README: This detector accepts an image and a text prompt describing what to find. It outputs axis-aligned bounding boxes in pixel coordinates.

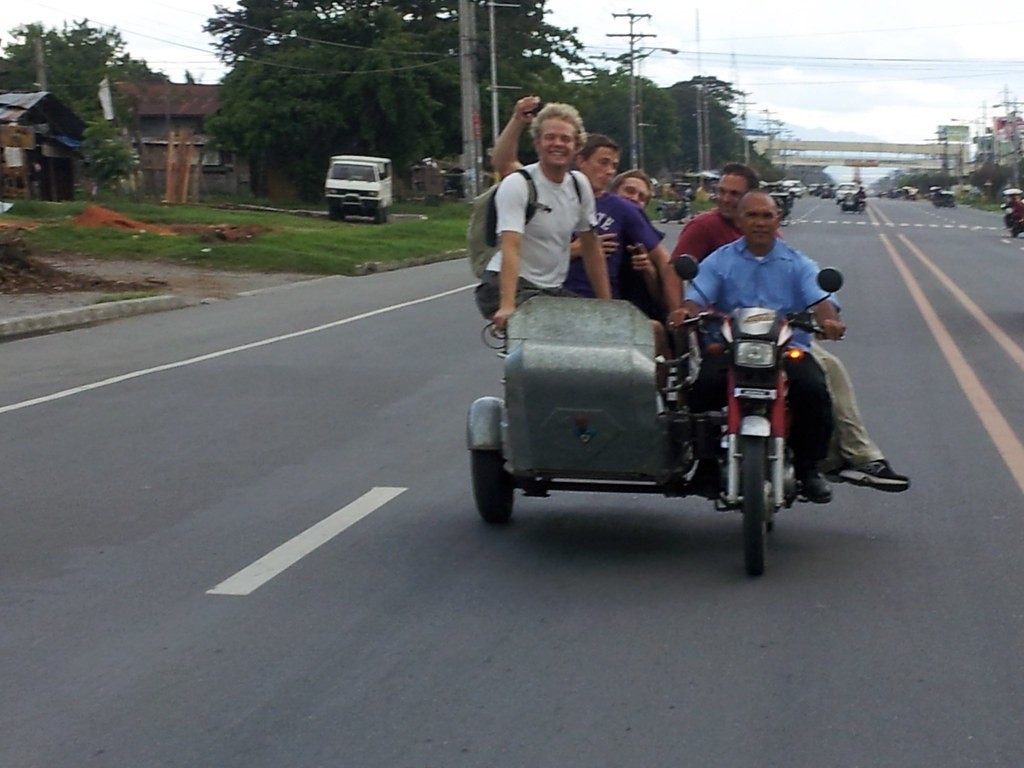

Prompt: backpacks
[467,169,582,280]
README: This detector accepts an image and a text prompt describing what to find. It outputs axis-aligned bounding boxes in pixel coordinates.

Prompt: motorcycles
[930,187,954,208]
[1000,190,1024,236]
[468,254,848,578]
[657,189,694,226]
[840,186,867,214]
[771,193,791,227]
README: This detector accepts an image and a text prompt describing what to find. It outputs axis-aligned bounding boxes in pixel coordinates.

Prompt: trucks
[326,156,392,223]
[682,173,719,203]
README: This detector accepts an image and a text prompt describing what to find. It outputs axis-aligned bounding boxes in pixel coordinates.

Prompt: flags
[997,116,1024,129]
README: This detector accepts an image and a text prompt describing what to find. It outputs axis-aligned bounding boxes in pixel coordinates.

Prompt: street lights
[636,48,679,170]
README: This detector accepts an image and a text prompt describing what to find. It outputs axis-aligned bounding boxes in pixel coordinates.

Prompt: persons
[1004,196,1024,227]
[569,170,662,296]
[668,164,914,486]
[489,99,682,314]
[665,191,846,505]
[475,104,665,414]
[853,186,866,203]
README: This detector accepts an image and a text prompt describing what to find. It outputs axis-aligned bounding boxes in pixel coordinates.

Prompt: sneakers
[838,458,911,493]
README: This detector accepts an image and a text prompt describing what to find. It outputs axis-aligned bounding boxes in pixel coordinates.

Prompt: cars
[788,186,802,197]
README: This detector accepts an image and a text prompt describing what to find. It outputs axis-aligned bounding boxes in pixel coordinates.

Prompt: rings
[669,321,674,325]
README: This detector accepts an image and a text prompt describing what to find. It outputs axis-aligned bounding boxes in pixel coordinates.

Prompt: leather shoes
[801,468,833,505]
